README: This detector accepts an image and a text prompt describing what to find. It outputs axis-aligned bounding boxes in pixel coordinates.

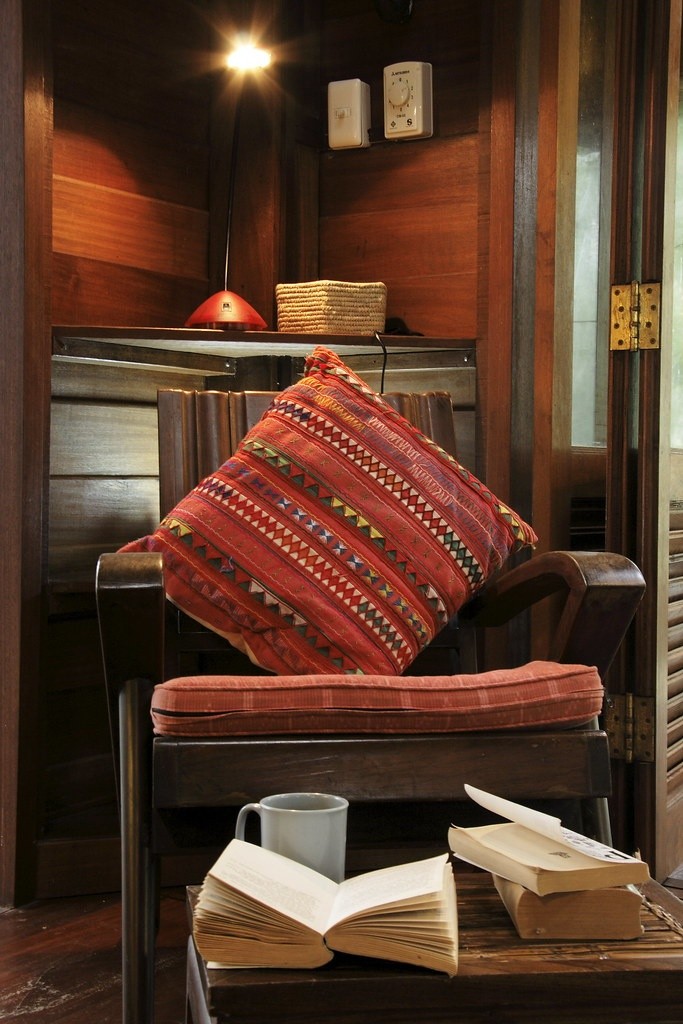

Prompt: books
[490,872,644,939]
[192,839,458,978]
[447,783,650,897]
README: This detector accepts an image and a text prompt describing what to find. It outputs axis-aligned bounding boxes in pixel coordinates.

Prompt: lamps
[178,20,284,334]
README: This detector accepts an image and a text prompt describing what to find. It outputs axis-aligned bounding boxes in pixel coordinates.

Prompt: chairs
[95,389,683,1024]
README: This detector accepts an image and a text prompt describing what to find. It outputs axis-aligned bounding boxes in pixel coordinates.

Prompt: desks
[184,861,683,1024]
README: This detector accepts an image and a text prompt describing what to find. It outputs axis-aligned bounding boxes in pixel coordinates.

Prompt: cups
[234,791,349,884]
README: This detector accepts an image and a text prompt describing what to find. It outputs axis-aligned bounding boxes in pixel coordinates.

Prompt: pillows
[116,344,542,682]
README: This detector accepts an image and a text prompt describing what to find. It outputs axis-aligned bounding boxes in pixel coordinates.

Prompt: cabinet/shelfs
[13,320,483,907]
